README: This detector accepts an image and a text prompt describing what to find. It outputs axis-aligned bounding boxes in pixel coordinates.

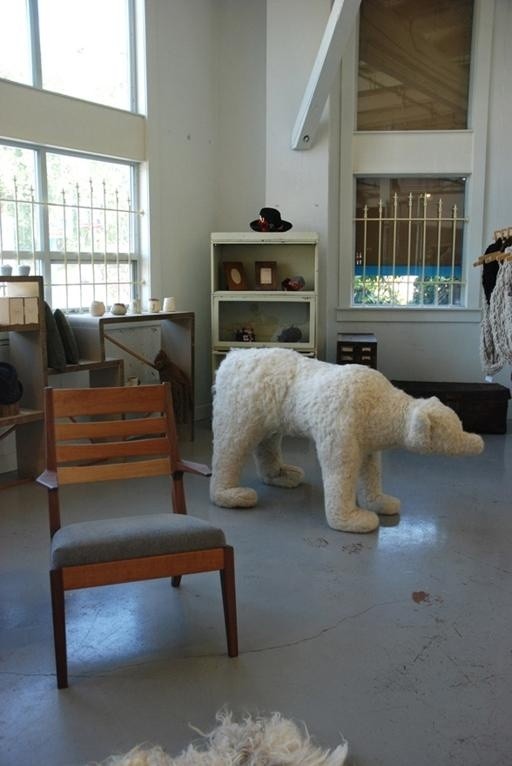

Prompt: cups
[147,298,161,313]
[129,299,141,314]
[110,302,128,316]
[88,301,106,318]
[0,264,13,276]
[17,266,31,276]
[128,376,138,387]
[163,297,177,313]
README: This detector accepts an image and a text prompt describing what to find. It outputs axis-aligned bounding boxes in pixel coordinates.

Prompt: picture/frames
[223,259,277,292]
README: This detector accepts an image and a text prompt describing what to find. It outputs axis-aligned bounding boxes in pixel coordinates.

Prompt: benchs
[383,382,512,438]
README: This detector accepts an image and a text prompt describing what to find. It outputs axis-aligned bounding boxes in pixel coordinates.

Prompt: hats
[249,207,293,232]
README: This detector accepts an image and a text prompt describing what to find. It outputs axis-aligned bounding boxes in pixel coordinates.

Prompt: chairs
[41,380,242,688]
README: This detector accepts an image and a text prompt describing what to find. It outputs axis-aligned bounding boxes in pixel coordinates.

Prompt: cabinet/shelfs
[0,274,198,494]
[208,229,322,402]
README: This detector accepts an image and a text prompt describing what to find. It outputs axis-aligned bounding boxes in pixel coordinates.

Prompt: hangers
[473,224,511,269]
